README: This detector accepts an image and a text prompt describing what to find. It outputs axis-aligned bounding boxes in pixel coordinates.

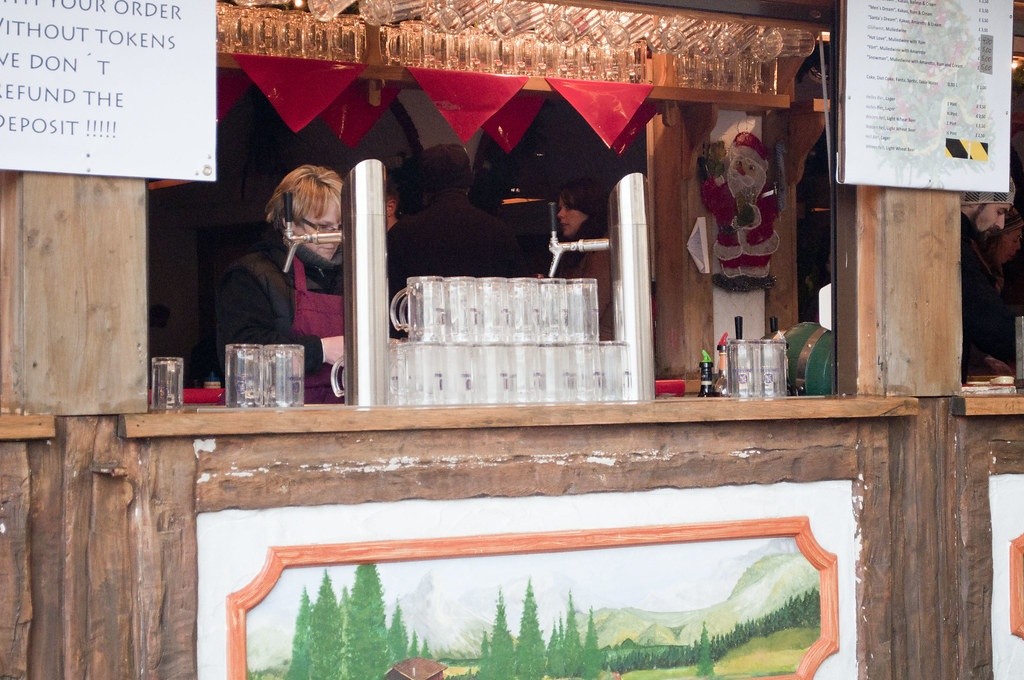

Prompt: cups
[661,15,709,55]
[553,8,601,47]
[445,276,476,341]
[358,0,426,27]
[379,20,647,84]
[493,1,558,41]
[729,339,787,397]
[507,277,539,342]
[264,344,304,407]
[693,22,734,58]
[330,341,629,404]
[762,27,814,57]
[539,278,565,342]
[566,278,599,341]
[152,357,184,410]
[307,0,358,22]
[216,2,366,64]
[672,55,764,95]
[477,277,511,341]
[712,23,760,60]
[655,379,686,397]
[225,344,263,408]
[606,11,655,50]
[390,276,446,341]
[438,0,505,35]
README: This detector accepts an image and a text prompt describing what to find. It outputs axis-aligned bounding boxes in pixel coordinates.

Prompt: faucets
[546,201,611,278]
[281,190,343,274]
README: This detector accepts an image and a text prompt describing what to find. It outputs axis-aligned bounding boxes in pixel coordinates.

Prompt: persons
[536,179,615,341]
[961,176,1015,384]
[976,205,1024,299]
[215,165,345,405]
[385,142,533,339]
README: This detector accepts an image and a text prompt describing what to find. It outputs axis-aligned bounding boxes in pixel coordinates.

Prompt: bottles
[760,316,786,339]
[713,345,728,397]
[698,362,714,397]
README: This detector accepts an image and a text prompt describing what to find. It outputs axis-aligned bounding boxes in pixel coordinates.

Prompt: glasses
[293,215,342,234]
